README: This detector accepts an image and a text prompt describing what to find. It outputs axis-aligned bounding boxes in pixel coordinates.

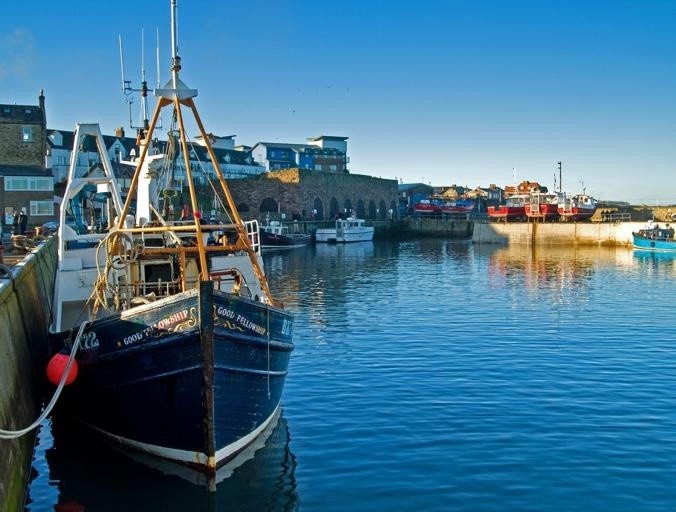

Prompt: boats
[631,248,676,263]
[414,189,601,221]
[629,213,676,250]
[26,425,305,512]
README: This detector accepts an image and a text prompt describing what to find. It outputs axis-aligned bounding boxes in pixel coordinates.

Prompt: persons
[11,210,19,236]
[18,211,28,233]
